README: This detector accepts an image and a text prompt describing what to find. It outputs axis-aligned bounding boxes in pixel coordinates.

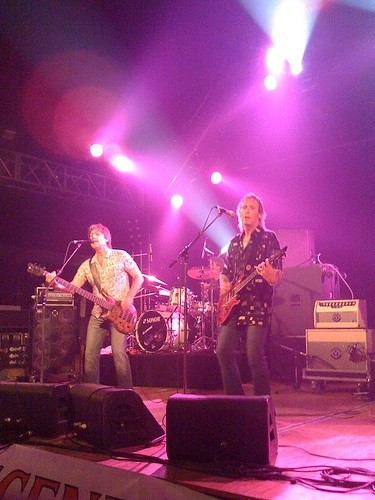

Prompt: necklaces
[243,237,248,248]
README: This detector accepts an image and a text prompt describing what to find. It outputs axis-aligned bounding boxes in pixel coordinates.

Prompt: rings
[259,268,262,271]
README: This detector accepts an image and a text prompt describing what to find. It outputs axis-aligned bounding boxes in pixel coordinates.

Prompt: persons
[45,224,145,388]
[220,193,283,416]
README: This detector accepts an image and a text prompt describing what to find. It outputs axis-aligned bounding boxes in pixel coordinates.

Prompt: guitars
[217,245,289,325]
[27,263,138,334]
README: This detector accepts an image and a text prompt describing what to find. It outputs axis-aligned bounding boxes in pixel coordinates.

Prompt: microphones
[74,239,93,243]
[149,244,153,261]
[215,204,235,217]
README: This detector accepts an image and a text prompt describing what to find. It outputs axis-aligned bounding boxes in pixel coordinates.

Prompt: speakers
[70,381,165,452]
[0,381,73,436]
[166,393,276,469]
[31,302,77,380]
[307,328,375,371]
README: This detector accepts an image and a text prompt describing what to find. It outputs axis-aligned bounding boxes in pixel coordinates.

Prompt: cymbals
[143,275,167,286]
[188,266,216,280]
[150,284,170,292]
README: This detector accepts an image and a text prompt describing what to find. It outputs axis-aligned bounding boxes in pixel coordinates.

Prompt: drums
[134,310,189,354]
[167,286,193,312]
[190,300,212,315]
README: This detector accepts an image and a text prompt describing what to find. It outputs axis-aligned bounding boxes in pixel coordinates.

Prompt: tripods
[188,281,218,353]
